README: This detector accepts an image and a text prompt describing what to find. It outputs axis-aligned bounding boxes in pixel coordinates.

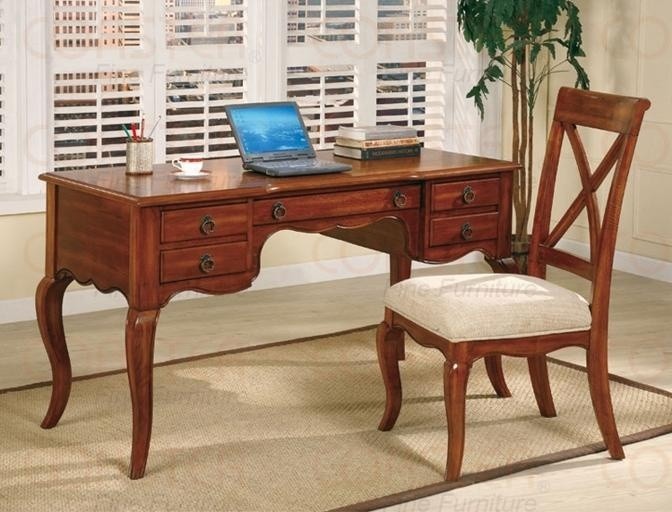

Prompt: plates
[172,171,210,180]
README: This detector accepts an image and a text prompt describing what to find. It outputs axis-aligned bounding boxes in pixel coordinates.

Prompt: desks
[37,145,525,479]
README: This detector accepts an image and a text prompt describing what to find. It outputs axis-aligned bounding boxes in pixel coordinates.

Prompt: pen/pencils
[122,113,161,142]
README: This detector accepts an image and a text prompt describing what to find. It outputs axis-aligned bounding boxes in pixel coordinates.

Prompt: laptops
[224,101,352,177]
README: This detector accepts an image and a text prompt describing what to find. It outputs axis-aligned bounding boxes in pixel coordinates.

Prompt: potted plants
[457,0,590,274]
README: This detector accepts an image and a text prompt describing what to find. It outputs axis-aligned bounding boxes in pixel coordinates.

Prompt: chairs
[376,88,650,484]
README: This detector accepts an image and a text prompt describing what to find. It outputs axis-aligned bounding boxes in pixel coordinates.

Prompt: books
[332,144,420,160]
[335,136,418,149]
[339,123,417,141]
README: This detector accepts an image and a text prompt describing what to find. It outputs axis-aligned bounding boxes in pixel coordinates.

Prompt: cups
[172,157,203,174]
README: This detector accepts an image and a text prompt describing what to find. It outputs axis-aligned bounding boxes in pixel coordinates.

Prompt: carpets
[0,324,672,512]
